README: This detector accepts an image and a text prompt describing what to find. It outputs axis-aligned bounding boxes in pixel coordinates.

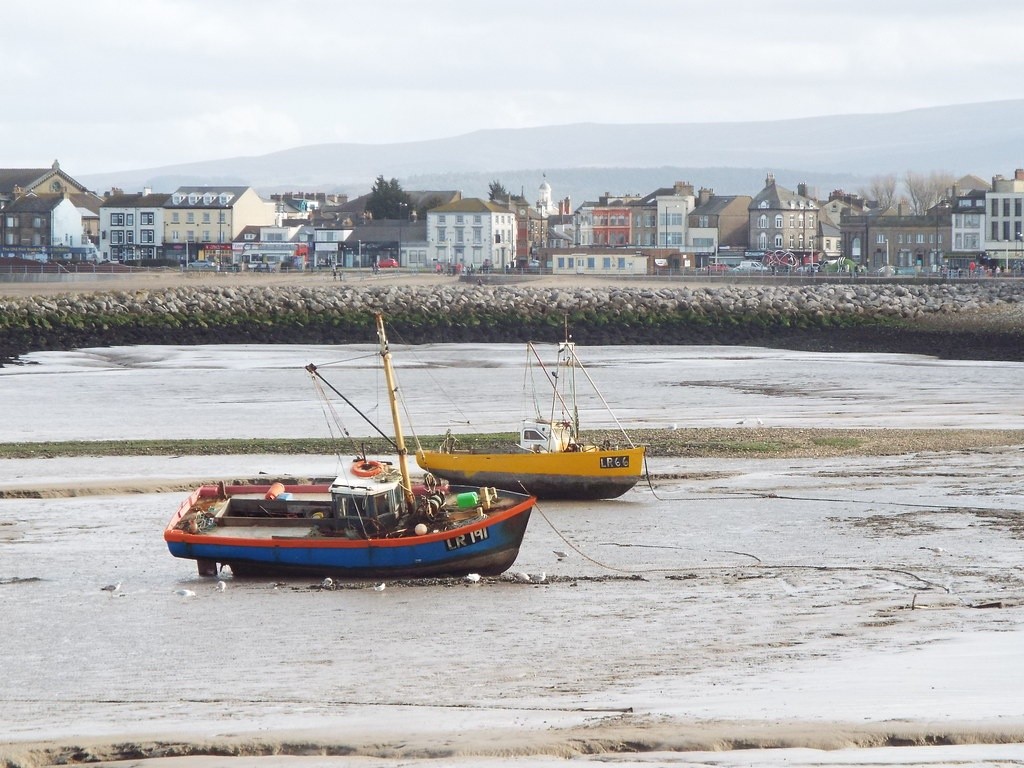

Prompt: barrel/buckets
[457,492,478,509]
[266,483,285,501]
[277,493,293,500]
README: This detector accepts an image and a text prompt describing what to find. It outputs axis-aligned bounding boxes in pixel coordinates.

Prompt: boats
[415,299,646,500]
[164,311,533,579]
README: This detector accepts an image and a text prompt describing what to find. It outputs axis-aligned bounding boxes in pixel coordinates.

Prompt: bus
[239,241,310,271]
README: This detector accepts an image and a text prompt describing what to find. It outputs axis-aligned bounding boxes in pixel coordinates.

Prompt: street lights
[393,202,408,268]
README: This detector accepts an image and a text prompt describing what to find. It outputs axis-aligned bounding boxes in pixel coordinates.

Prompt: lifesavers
[350,461,383,476]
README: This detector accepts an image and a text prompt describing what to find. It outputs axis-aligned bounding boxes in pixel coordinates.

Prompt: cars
[733,261,771,272]
[376,257,399,268]
[705,262,731,271]
